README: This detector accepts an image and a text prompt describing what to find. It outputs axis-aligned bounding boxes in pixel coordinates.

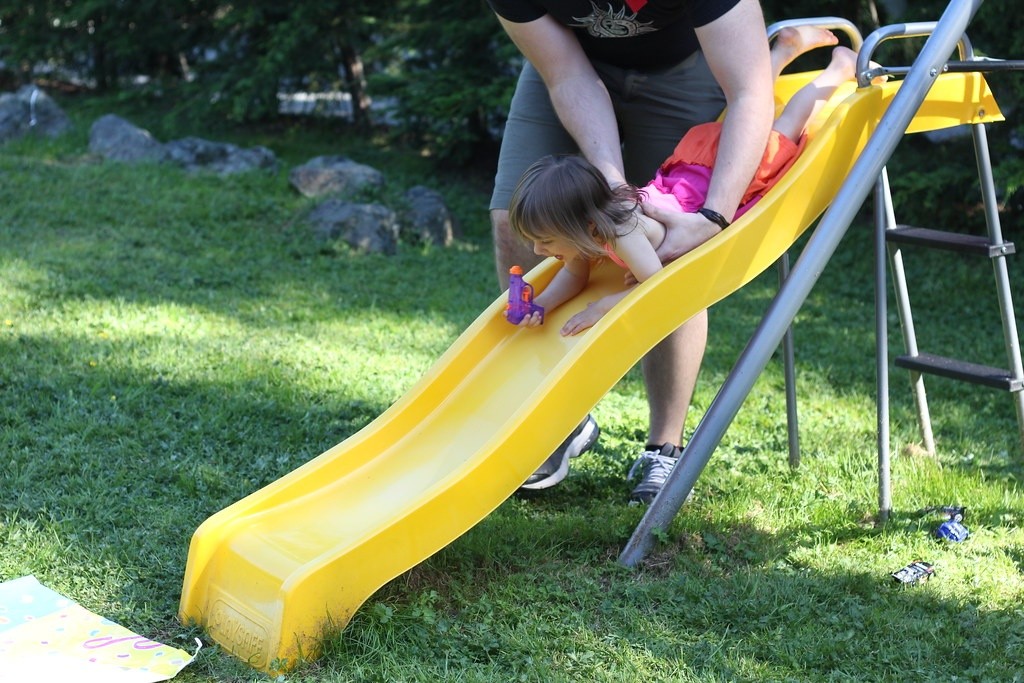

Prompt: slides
[176,65,1007,675]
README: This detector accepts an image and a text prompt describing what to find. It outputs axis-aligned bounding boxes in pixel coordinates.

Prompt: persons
[516,25,888,337]
[490,0,775,507]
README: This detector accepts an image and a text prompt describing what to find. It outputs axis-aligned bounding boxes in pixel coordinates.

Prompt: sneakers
[519,411,602,491]
[626,441,696,507]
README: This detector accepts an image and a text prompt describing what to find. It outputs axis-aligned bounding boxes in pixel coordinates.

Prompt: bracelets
[697,208,730,230]
